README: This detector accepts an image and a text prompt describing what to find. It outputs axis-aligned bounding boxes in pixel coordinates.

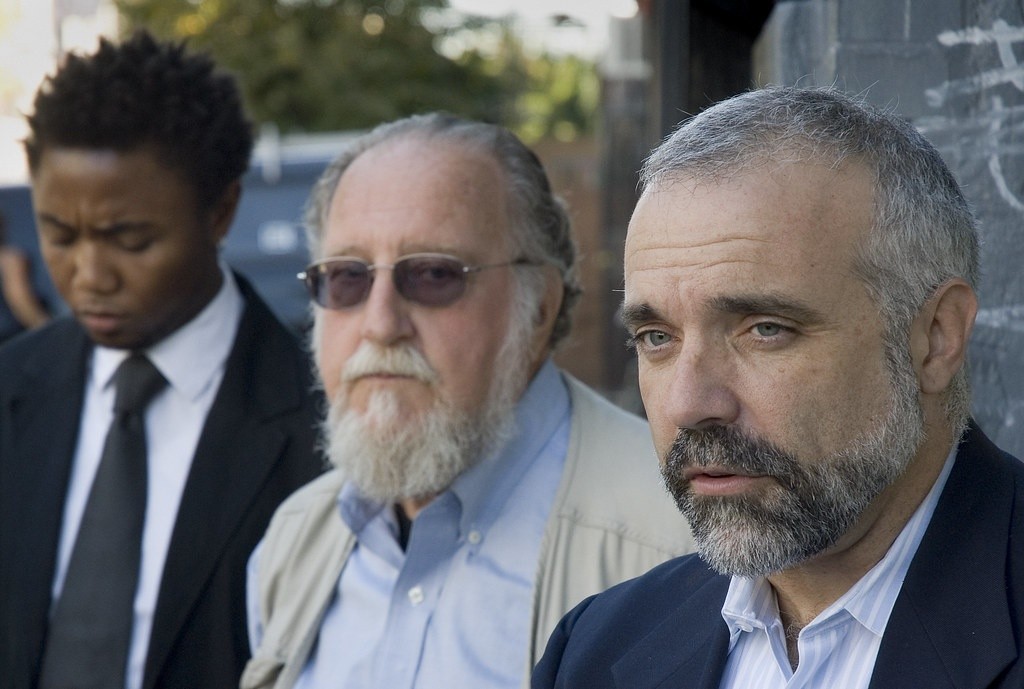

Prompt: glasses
[300,254,529,310]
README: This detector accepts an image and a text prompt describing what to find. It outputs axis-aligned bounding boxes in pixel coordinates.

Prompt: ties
[37,351,169,689]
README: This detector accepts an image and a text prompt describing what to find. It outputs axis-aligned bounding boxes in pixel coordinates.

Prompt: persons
[532,87,1024,689]
[0,30,332,689]
[240,111,700,689]
